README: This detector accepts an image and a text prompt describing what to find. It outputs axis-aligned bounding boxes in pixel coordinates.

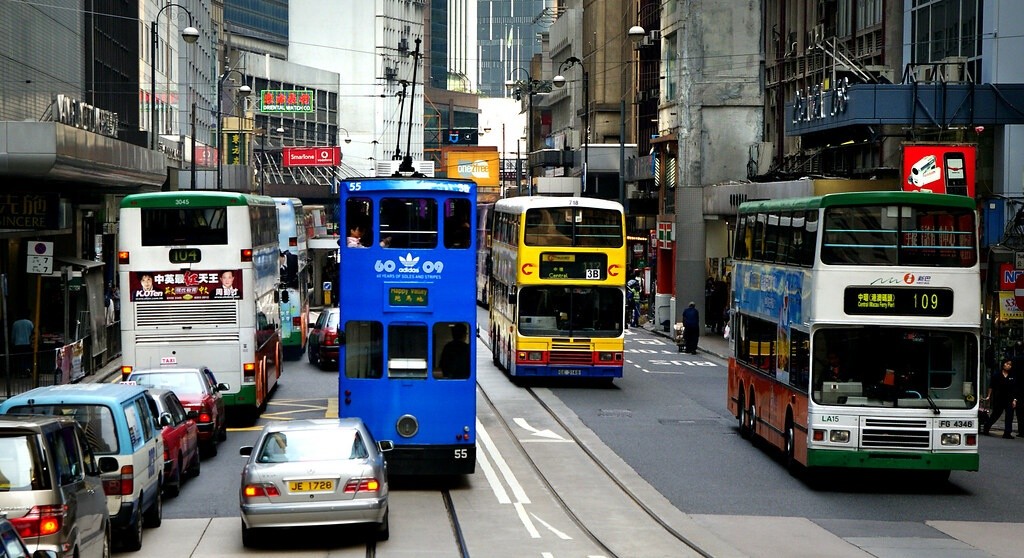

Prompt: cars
[307,307,340,370]
[146,388,202,500]
[127,365,230,459]
[0,514,59,558]
[238,418,395,552]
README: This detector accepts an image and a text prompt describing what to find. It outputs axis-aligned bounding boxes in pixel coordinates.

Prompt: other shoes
[692,351,697,355]
[636,325,643,328]
[26,369,31,377]
[684,349,691,353]
[1002,430,1014,439]
[630,324,635,327]
[984,424,991,436]
[21,374,28,377]
[1016,433,1024,436]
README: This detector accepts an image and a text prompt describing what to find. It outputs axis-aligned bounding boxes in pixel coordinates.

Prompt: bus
[727,192,982,487]
[489,195,626,388]
[118,191,288,426]
[206,197,314,360]
[338,176,478,472]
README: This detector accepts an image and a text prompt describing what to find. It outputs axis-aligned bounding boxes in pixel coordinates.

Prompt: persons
[439,324,470,379]
[983,357,1024,439]
[683,301,699,355]
[11,319,34,377]
[705,276,729,332]
[105,284,120,326]
[347,223,392,248]
[133,272,167,301]
[209,270,242,299]
[626,286,644,328]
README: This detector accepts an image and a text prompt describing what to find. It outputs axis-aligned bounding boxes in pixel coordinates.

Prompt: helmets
[628,279,636,286]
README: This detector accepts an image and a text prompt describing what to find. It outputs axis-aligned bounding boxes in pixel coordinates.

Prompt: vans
[0,413,119,558]
[0,384,173,553]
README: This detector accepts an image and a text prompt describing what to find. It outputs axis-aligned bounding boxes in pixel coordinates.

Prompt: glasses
[465,333,468,335]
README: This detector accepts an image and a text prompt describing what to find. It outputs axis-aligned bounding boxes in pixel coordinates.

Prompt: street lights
[505,68,533,153]
[151,4,200,150]
[261,117,285,195]
[217,70,252,191]
[553,56,589,196]
[332,128,351,192]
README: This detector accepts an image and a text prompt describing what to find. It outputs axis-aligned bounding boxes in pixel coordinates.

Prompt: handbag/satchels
[725,325,730,339]
[979,399,990,424]
